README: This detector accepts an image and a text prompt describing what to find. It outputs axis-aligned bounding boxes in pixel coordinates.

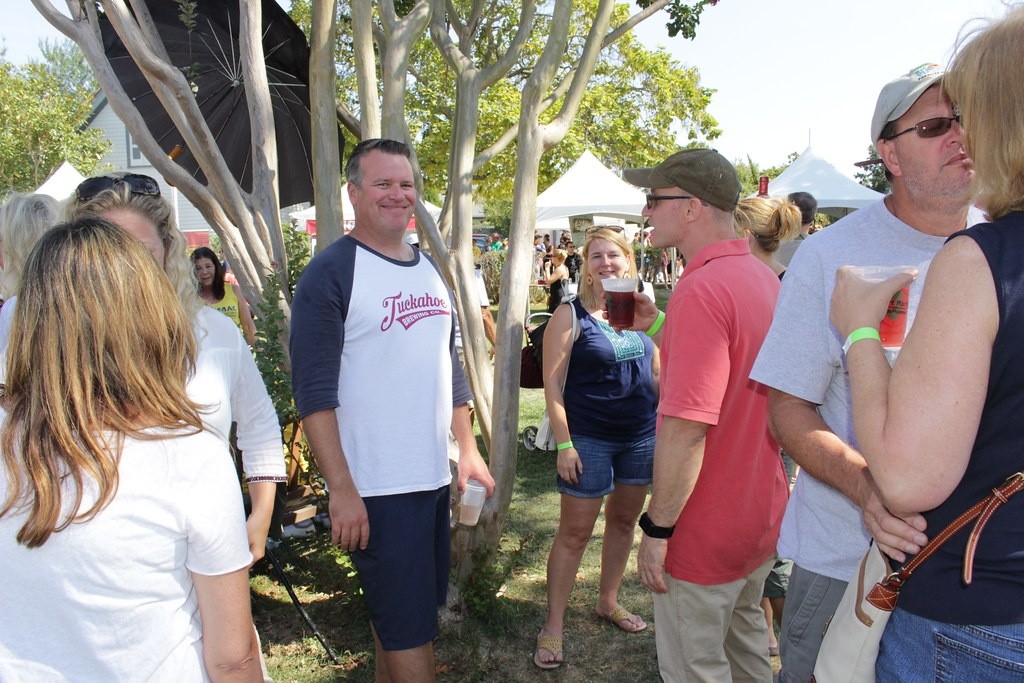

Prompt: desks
[530,284,550,287]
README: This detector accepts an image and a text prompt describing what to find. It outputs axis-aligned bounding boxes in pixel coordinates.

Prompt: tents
[291,182,443,257]
[743,146,887,218]
[534,149,675,293]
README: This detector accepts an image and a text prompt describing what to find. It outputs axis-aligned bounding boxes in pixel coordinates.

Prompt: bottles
[575,270,579,284]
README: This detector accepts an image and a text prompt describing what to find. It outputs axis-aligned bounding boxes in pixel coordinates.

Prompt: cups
[849,266,910,348]
[601,278,638,327]
[538,277,543,284]
[460,480,486,526]
[569,278,572,283]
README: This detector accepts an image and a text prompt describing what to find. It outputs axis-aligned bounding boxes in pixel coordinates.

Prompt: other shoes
[768,642,779,656]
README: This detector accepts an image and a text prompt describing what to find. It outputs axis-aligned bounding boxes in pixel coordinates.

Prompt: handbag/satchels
[812,540,901,683]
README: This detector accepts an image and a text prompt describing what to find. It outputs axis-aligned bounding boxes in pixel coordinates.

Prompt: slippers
[534,629,564,669]
[611,606,648,631]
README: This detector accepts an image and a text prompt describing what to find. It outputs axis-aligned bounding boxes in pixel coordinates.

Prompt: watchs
[639,512,675,538]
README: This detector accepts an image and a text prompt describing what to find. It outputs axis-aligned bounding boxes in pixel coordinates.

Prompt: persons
[534,226,661,668]
[637,148,791,683]
[0,174,289,569]
[734,192,817,281]
[190,247,263,353]
[0,218,274,683]
[545,248,569,320]
[472,230,686,290]
[829,5,1024,683]
[289,138,496,682]
[748,62,991,683]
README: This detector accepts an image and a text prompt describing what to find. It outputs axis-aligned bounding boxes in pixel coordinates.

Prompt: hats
[871,62,968,148]
[624,145,742,210]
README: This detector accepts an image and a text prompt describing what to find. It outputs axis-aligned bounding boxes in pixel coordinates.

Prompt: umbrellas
[97,0,345,209]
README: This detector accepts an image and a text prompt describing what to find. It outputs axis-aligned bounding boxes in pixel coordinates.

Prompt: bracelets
[842,327,881,357]
[557,441,573,451]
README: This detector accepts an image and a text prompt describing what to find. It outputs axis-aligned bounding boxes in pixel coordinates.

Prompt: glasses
[584,225,625,240]
[568,245,575,248]
[887,114,962,138]
[647,195,708,210]
[76,172,162,203]
